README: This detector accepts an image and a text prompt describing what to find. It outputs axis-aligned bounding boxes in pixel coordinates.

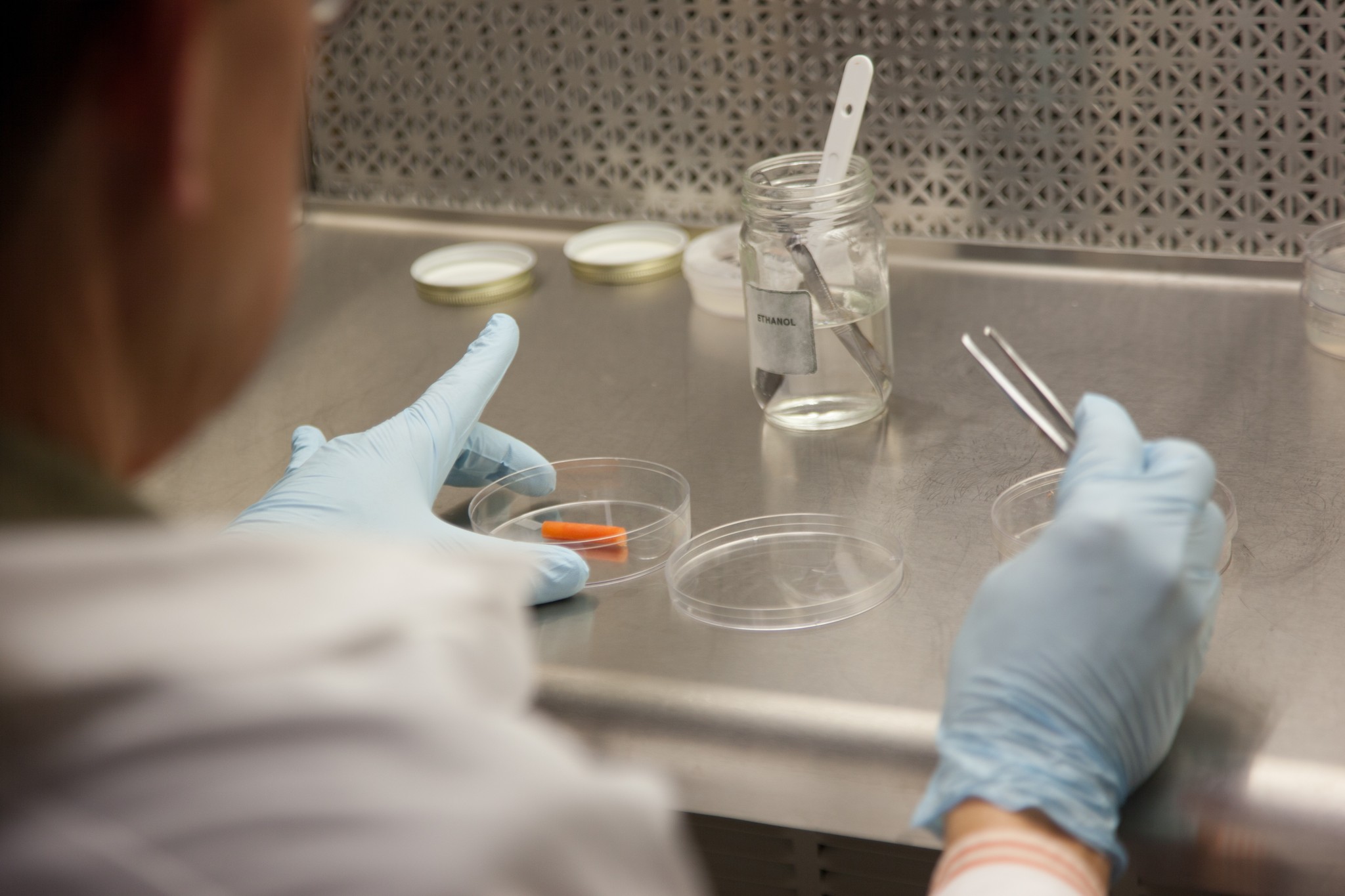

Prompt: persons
[0,0,1231,896]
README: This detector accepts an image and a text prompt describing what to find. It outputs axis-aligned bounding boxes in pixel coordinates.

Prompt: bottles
[737,150,895,432]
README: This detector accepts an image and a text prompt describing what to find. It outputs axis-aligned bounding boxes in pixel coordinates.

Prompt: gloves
[228,313,591,606]
[911,392,1228,888]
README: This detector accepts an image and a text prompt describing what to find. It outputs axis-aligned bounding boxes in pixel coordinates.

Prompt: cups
[1300,218,1345,362]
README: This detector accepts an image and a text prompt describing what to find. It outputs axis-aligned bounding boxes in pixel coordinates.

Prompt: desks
[132,214,1345,896]
